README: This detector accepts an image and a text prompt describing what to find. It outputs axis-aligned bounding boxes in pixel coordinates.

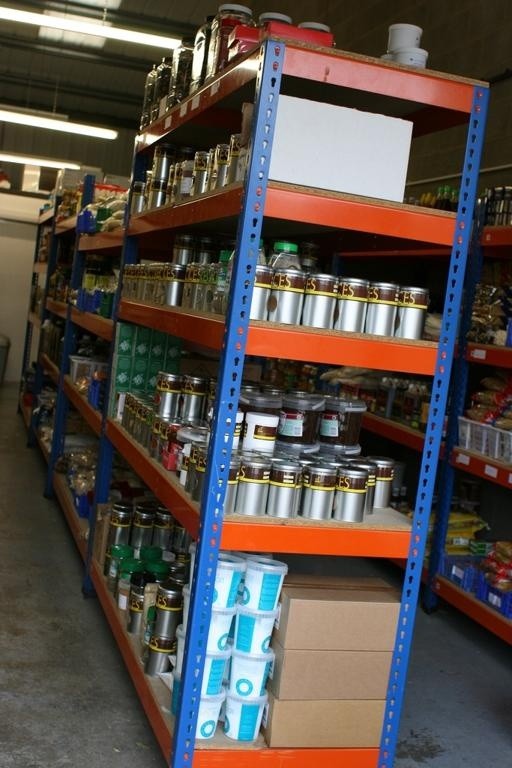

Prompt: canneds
[130,133,240,216]
[139,3,331,130]
[171,552,289,742]
[120,235,430,341]
[105,478,193,673]
[115,369,393,523]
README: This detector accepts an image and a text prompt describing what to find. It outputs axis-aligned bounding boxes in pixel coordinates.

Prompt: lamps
[0,148,81,174]
[0,103,120,143]
[1,6,183,51]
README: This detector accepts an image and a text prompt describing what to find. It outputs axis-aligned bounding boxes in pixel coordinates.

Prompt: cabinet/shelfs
[331,220,512,647]
[13,174,125,570]
[89,42,498,768]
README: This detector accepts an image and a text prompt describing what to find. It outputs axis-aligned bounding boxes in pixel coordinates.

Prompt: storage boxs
[266,91,416,204]
[259,574,402,748]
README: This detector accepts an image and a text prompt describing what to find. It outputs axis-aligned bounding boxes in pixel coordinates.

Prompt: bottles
[214,235,305,317]
[402,184,460,215]
